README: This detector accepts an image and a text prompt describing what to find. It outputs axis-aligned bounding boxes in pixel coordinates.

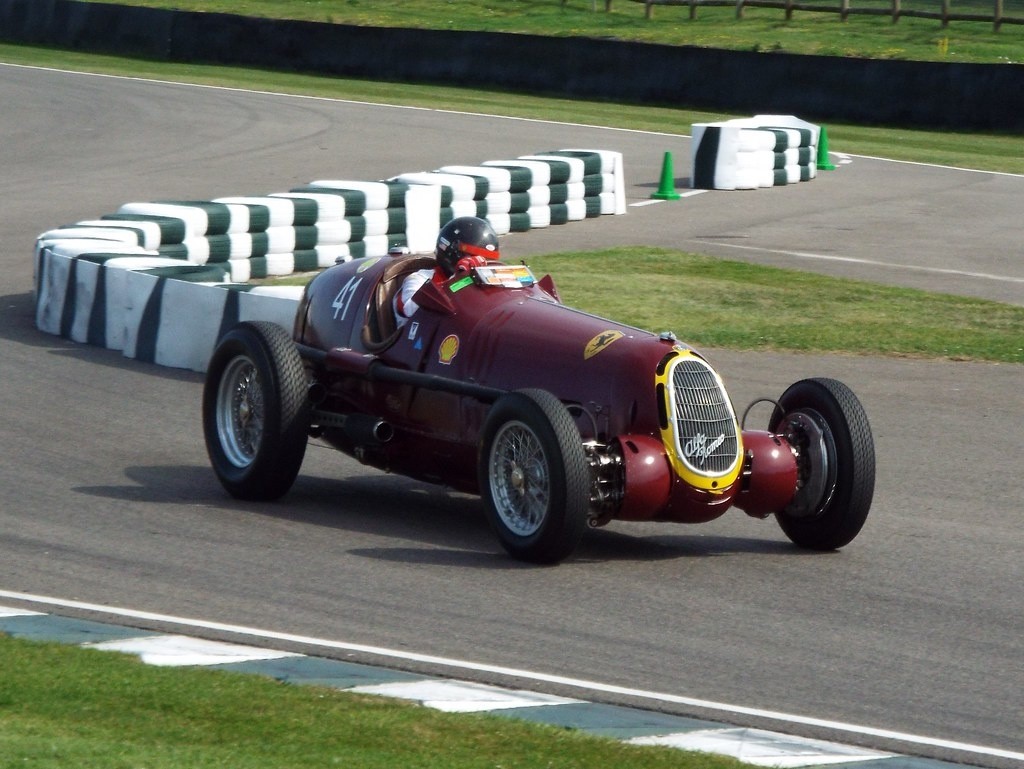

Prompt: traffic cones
[814,125,836,170]
[650,151,683,201]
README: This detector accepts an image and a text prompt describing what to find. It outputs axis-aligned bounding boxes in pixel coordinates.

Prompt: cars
[199,241,878,568]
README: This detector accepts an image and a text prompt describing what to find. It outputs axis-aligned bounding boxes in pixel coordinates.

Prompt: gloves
[455,256,488,278]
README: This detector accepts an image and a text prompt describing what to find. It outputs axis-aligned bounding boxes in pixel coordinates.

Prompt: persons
[392,217,501,330]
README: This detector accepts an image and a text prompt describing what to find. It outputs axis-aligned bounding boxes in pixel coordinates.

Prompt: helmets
[436,217,499,275]
